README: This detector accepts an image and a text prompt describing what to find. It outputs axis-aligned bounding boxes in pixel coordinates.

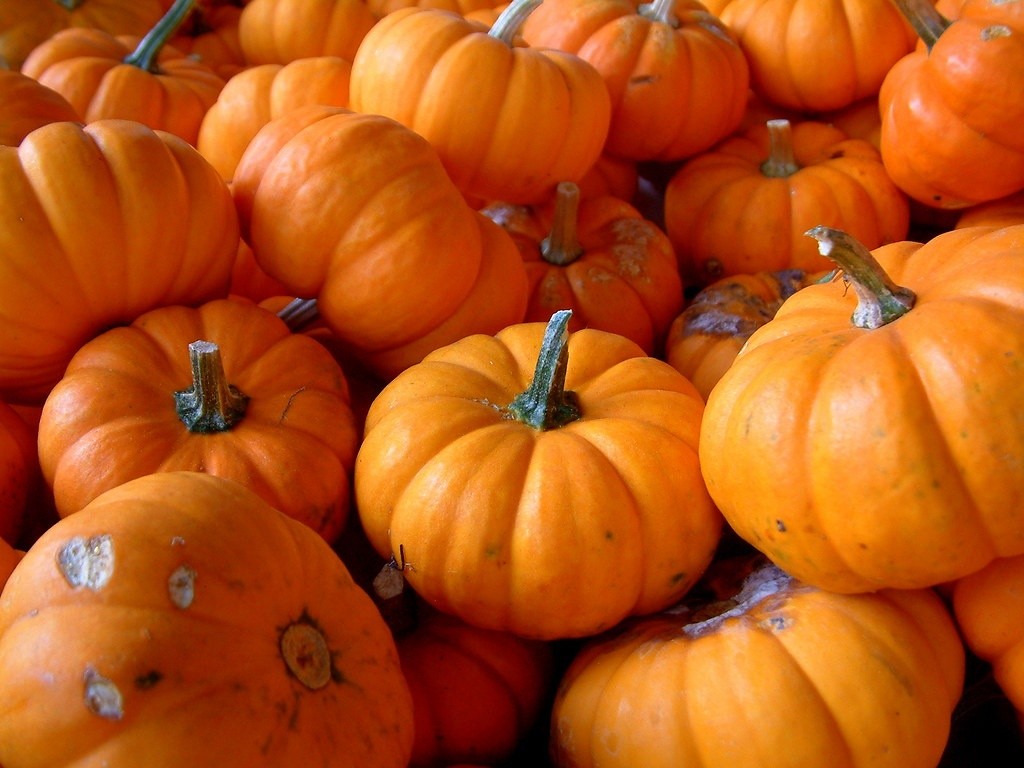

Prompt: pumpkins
[1,0,1023,768]
